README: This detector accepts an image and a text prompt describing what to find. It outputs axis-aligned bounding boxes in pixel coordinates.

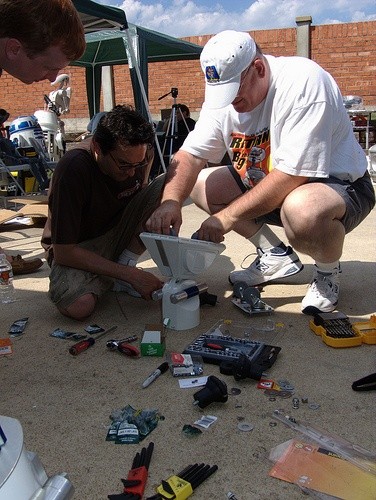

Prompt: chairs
[0,135,57,209]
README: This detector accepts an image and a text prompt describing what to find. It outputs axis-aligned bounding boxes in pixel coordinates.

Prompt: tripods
[158,88,209,177]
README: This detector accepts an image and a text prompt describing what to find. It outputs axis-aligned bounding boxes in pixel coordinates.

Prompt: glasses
[108,153,149,170]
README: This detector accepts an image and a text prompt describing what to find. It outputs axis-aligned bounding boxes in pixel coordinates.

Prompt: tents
[68,22,203,123]
[72,0,166,174]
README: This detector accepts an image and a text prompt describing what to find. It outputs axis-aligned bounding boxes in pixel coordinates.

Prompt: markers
[141,361,170,389]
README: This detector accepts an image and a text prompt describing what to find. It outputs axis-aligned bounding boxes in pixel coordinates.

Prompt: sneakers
[110,277,141,298]
[299,262,343,315]
[227,246,304,286]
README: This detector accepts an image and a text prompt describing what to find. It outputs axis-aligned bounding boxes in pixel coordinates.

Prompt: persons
[150,104,196,180]
[0,109,51,191]
[55,129,66,158]
[40,104,167,318]
[146,30,376,315]
[0,1,85,84]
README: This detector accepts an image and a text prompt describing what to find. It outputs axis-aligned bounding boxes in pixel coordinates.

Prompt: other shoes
[9,253,44,276]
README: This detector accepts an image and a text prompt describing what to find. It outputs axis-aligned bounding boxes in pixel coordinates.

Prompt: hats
[200,30,256,109]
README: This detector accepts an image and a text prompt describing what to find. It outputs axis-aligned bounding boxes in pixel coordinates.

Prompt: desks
[347,106,376,149]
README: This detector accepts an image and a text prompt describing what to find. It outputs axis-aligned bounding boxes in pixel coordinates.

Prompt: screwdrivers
[68,325,118,357]
